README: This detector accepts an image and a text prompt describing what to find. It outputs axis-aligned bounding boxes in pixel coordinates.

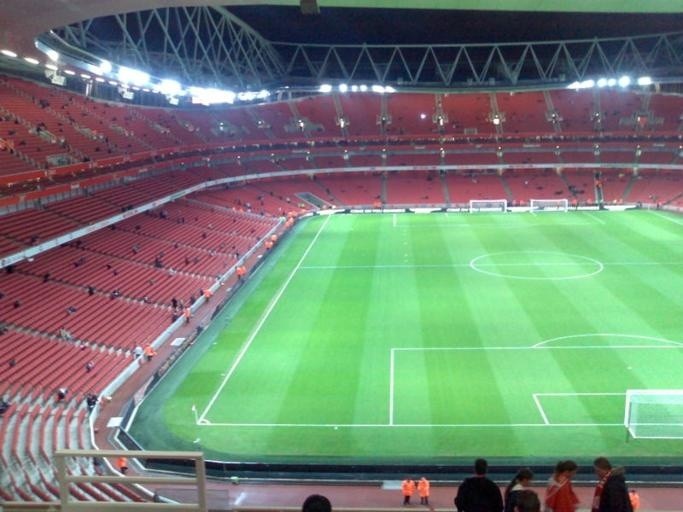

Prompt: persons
[627,488,639,511]
[86,389,97,412]
[298,494,332,512]
[454,459,506,511]
[196,189,307,305]
[114,457,130,474]
[143,341,154,361]
[588,457,634,512]
[513,491,542,511]
[132,342,142,358]
[169,296,191,326]
[503,465,532,511]
[545,459,580,511]
[401,475,416,506]
[416,475,432,506]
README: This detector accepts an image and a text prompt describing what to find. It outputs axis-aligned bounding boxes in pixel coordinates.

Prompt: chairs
[0,71,285,512]
